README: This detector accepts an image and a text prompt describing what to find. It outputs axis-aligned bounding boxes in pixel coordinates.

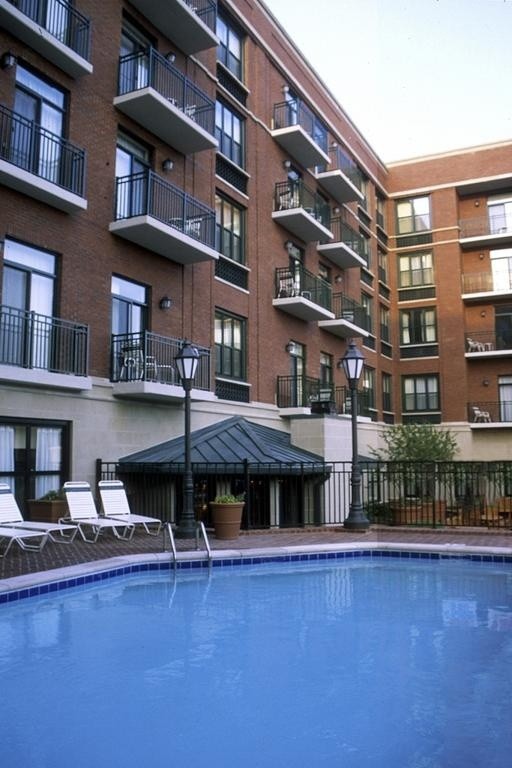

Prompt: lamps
[286,339,295,352]
[158,294,173,309]
[167,49,175,63]
[163,156,174,173]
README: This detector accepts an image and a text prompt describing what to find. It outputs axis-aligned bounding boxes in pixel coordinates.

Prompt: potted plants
[365,424,461,524]
[210,493,245,540]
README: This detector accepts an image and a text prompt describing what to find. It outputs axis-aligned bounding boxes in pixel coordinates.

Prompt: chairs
[279,191,299,210]
[473,407,491,422]
[278,277,294,298]
[0,480,162,559]
[170,217,202,239]
[118,347,175,384]
[181,105,196,121]
[343,396,351,414]
[309,388,333,405]
[467,337,485,352]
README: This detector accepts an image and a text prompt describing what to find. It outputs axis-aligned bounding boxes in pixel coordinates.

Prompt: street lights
[340,343,372,528]
[173,338,203,539]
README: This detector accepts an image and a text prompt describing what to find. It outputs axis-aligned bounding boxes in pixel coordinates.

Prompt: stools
[484,343,494,351]
[168,98,178,107]
[300,290,311,300]
